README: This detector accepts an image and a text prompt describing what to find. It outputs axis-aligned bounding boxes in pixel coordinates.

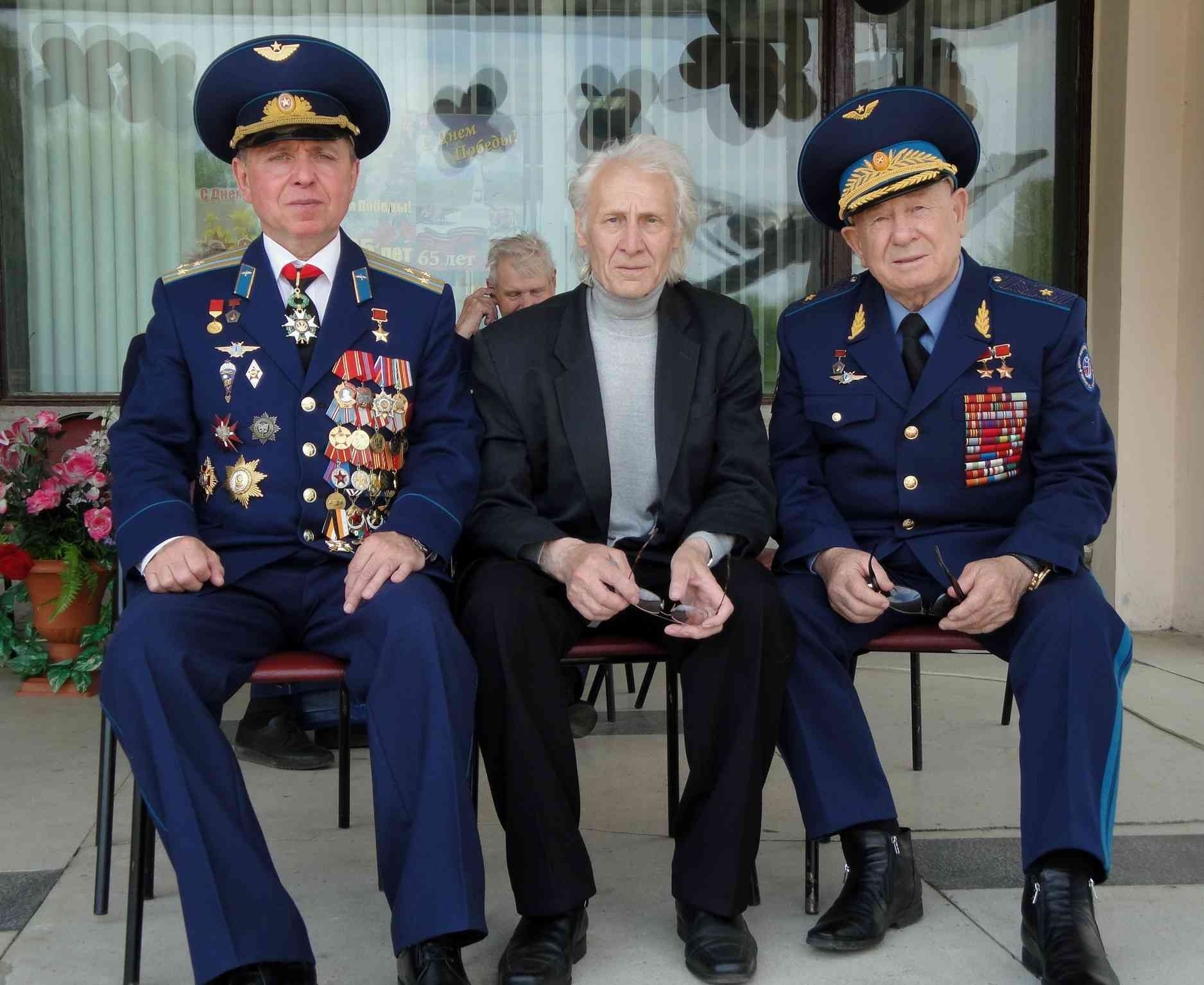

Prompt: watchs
[1011,553,1053,591]
[398,531,429,555]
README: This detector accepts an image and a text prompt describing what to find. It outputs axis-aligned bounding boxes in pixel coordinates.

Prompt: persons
[772,86,1132,983]
[456,133,779,984]
[456,233,601,737]
[97,35,489,984]
[234,675,367,770]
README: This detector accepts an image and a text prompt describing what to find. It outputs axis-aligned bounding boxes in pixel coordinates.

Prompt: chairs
[94,551,1017,985]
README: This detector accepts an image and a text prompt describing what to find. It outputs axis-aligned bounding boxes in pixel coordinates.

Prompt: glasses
[615,529,730,628]
[861,540,966,622]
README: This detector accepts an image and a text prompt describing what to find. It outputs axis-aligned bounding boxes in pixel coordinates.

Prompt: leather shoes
[395,932,471,985]
[1019,867,1122,985]
[673,899,758,984]
[234,712,335,771]
[497,899,589,985]
[806,824,923,950]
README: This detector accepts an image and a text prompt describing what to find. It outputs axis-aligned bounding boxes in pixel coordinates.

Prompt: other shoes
[564,699,598,738]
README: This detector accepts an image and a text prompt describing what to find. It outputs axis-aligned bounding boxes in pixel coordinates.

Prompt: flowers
[0,401,118,694]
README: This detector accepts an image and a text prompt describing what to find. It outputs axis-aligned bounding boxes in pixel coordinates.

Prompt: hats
[191,34,391,166]
[794,86,981,234]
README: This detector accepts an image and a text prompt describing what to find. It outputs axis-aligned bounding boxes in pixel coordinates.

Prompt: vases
[16,559,111,699]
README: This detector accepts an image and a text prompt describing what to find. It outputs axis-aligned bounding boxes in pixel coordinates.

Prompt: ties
[281,261,325,373]
[897,312,933,391]
